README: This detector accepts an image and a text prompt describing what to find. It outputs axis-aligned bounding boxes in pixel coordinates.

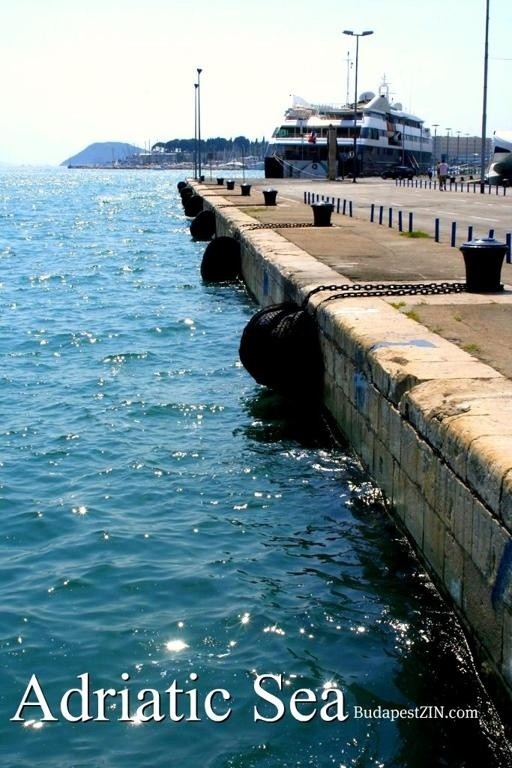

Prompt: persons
[436,159,448,192]
[427,165,433,181]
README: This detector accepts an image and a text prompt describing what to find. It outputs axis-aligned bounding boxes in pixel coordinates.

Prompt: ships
[265,54,433,178]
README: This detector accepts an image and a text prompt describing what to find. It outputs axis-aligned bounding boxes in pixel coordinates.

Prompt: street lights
[473,137,476,161]
[445,128,452,162]
[400,118,407,165]
[344,31,373,181]
[419,120,425,163]
[191,68,204,180]
[456,131,460,162]
[464,133,468,163]
[432,124,437,176]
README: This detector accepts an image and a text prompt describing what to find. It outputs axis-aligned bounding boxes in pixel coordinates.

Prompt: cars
[448,165,476,176]
[379,167,414,179]
